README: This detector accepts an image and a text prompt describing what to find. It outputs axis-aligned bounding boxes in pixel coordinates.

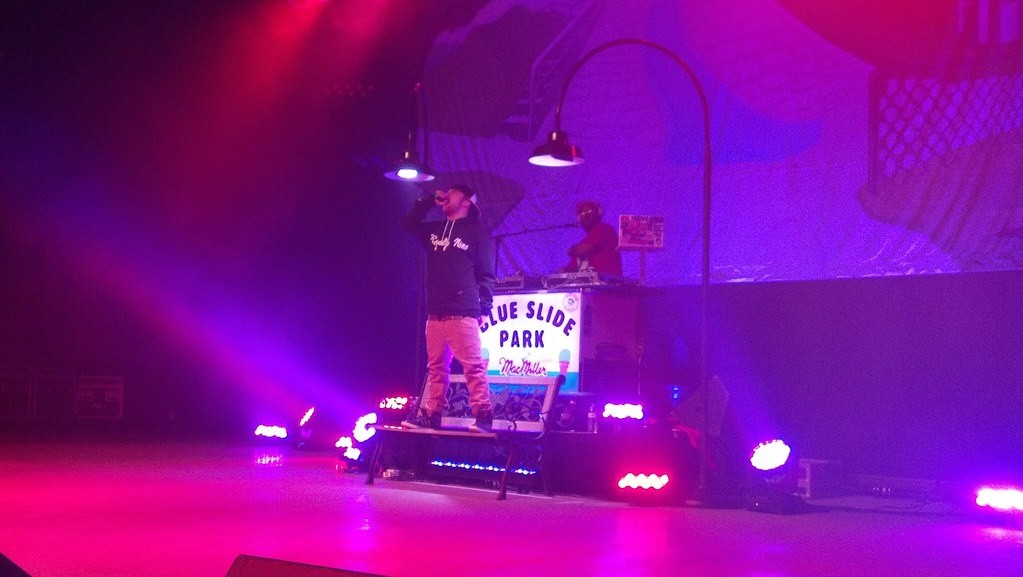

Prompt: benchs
[363,369,564,501]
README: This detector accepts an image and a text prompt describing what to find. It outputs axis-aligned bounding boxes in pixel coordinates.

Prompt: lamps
[528,39,732,509]
[383,83,437,386]
[747,438,812,515]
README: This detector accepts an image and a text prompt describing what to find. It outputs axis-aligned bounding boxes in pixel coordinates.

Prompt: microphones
[413,182,447,202]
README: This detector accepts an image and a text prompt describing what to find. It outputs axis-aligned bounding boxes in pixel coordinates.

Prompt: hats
[450,184,481,221]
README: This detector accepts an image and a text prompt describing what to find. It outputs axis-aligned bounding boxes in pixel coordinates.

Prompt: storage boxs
[72,376,140,422]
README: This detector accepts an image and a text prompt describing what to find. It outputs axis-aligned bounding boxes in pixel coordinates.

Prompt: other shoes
[468,423,493,433]
[401,407,441,430]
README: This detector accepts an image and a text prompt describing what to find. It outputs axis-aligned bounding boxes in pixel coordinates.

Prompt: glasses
[579,208,597,216]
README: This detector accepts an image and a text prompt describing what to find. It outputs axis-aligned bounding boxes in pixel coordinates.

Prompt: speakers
[539,429,700,508]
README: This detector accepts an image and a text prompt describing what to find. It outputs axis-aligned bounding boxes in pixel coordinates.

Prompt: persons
[403,184,492,433]
[567,199,622,279]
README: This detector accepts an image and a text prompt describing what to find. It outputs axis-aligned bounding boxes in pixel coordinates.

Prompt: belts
[428,314,464,321]
